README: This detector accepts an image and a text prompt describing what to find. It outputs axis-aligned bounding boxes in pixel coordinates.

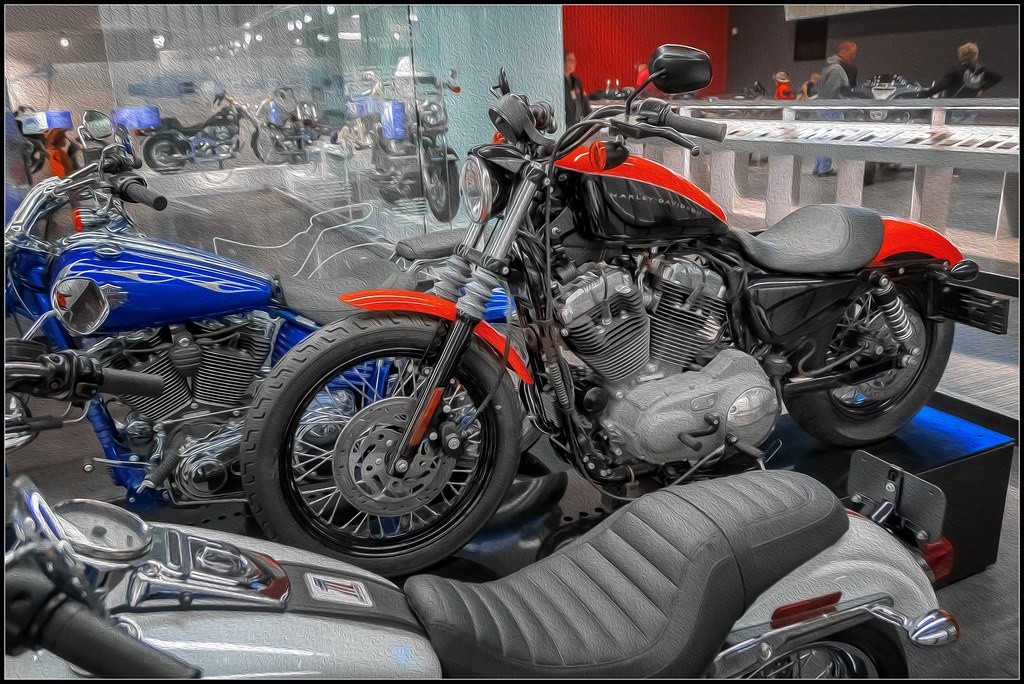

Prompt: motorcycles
[234,42,980,582]
[0,273,961,682]
[343,55,466,225]
[842,72,942,186]
[0,71,523,510]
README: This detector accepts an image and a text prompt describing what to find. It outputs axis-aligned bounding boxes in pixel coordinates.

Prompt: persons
[771,41,1004,185]
[563,52,592,127]
[633,60,651,96]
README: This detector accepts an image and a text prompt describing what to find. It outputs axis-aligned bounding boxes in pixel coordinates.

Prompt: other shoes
[818,167,838,179]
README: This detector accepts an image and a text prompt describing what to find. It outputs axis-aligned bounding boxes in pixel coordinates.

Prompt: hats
[772,72,790,83]
